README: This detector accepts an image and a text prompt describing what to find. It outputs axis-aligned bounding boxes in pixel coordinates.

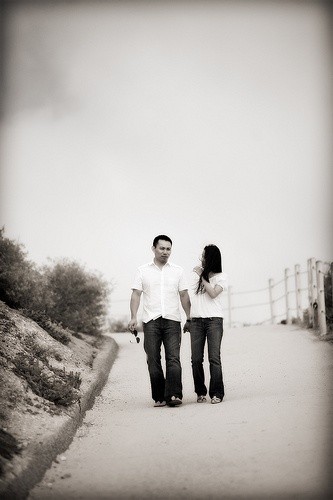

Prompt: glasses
[129,328,140,344]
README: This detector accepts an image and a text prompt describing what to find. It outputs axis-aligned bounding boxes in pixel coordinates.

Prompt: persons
[128,235,191,407]
[189,244,226,404]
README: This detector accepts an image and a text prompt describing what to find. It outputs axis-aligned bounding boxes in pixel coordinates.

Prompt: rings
[195,270,197,271]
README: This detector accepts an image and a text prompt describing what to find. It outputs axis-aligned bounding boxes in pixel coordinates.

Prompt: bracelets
[186,320,191,322]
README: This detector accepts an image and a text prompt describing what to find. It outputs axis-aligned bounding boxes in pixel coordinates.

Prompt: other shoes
[211,396,221,404]
[153,396,182,407]
[197,395,207,402]
